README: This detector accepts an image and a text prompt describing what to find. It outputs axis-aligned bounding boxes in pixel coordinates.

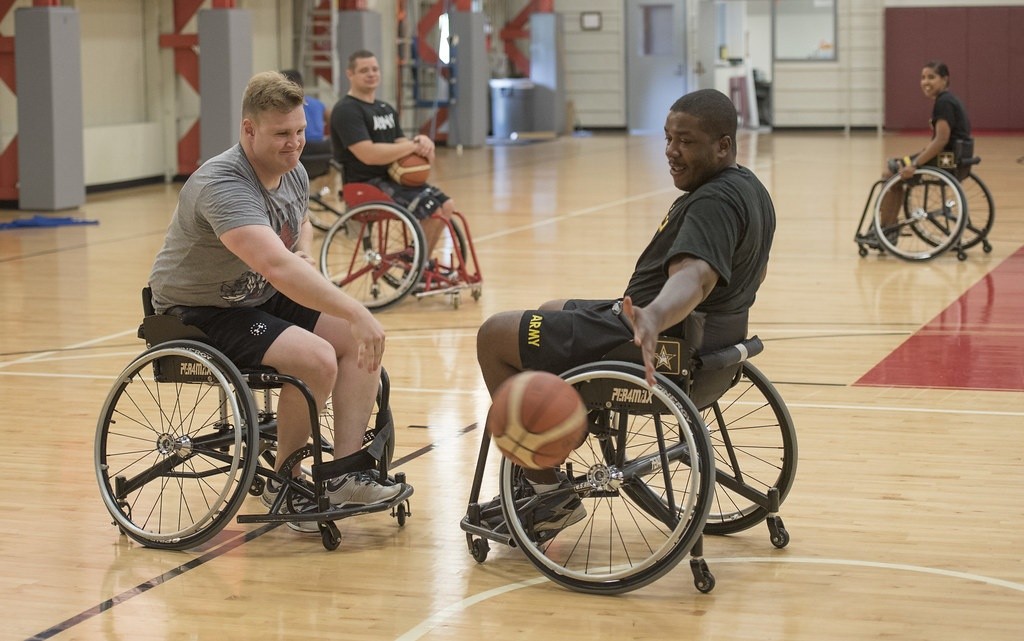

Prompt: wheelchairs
[92,284,414,552]
[299,140,348,235]
[319,164,485,314]
[459,312,799,597]
[854,140,996,265]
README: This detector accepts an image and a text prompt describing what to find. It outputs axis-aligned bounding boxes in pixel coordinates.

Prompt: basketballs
[387,153,430,187]
[487,369,588,471]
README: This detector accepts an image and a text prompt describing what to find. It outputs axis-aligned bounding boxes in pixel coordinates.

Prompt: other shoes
[400,261,458,289]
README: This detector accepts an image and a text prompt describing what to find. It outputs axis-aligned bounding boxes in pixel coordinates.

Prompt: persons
[329,50,457,288]
[477,89,776,535]
[855,62,973,252]
[147,70,402,540]
[279,68,331,143]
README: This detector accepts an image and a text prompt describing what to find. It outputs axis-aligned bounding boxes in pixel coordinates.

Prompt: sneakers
[324,471,406,512]
[258,476,326,533]
[475,471,586,538]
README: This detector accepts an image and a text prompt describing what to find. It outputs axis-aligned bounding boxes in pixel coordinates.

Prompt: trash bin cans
[489,77,534,138]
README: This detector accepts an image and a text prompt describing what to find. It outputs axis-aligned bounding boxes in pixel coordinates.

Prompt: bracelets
[911,161,921,169]
